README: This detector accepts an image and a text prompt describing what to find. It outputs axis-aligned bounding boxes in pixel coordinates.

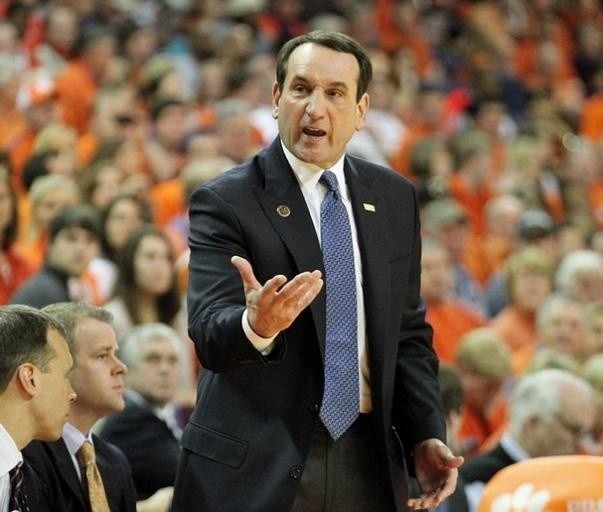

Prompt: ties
[8,464,32,512]
[75,440,112,512]
[313,170,361,443]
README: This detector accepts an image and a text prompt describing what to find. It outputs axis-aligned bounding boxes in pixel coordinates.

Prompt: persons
[0,2,601,465]
[0,302,78,511]
[32,297,140,512]
[84,318,198,512]
[418,363,472,512]
[445,369,602,486]
[160,25,469,512]
